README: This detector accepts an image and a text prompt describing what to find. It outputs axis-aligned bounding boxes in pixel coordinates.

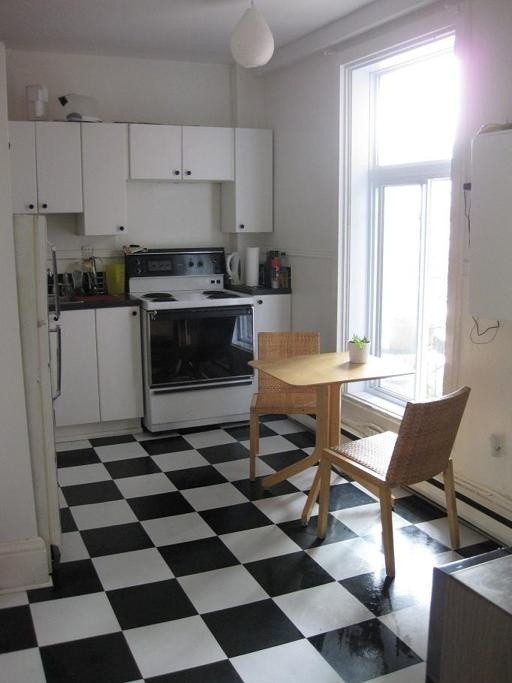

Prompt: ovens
[146,303,255,393]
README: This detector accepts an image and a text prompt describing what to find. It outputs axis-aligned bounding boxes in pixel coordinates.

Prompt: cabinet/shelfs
[225,296,291,392]
[9,121,274,236]
[48,307,145,443]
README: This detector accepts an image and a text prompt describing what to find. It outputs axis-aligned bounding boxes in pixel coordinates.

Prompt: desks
[248,350,415,527]
[316,386,471,578]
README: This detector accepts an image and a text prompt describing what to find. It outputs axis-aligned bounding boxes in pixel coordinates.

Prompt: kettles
[226,251,245,286]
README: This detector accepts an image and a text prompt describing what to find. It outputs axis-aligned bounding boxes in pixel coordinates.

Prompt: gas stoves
[128,289,253,312]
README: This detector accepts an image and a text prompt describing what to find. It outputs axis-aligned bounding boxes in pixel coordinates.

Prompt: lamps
[229,0,274,68]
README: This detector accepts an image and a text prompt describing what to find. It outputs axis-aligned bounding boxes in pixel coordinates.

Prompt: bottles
[81,244,98,296]
[263,251,289,292]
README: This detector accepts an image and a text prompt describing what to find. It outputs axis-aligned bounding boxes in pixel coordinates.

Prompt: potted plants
[347,334,370,364]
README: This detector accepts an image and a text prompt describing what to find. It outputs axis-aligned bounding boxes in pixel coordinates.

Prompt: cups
[105,263,125,295]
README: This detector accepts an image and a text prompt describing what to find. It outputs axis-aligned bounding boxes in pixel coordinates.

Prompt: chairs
[249,331,319,481]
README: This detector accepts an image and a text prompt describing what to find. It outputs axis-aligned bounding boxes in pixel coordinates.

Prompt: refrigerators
[12,214,62,574]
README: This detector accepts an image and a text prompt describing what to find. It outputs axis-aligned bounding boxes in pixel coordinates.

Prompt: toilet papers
[244,246,260,287]
